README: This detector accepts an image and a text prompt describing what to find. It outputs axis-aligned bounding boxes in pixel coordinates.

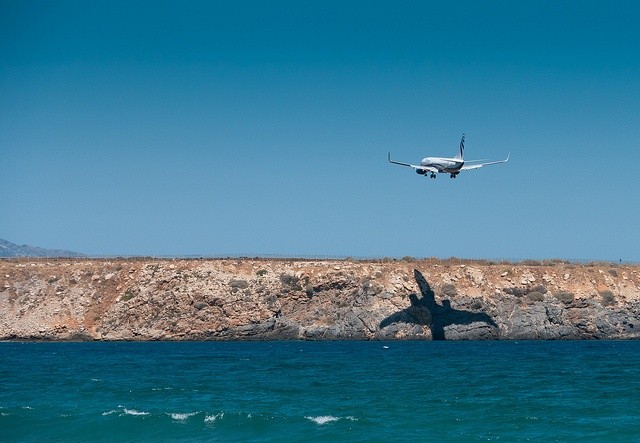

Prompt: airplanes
[388,132,510,179]
